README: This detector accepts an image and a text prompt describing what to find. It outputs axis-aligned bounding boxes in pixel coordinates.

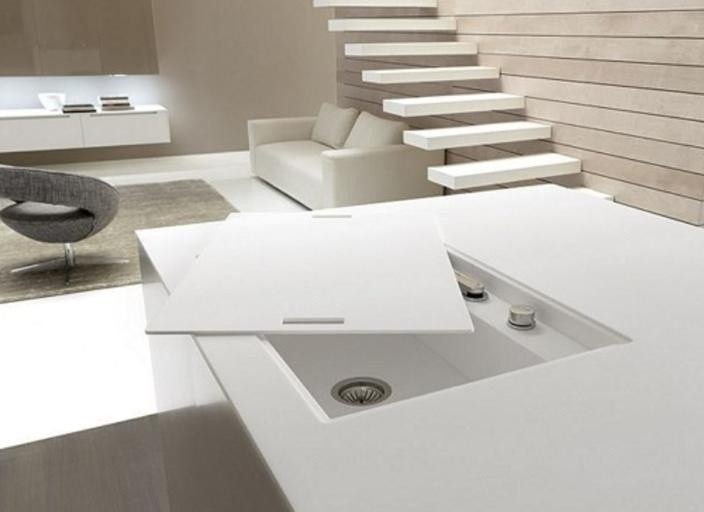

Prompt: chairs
[0,164,130,286]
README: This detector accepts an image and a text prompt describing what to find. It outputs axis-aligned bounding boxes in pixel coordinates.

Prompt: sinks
[255,247,640,433]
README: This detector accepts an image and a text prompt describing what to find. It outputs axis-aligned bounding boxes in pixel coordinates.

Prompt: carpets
[0,179,240,304]
[0,400,294,512]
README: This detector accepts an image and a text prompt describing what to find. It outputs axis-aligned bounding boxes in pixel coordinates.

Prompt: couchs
[246,102,444,210]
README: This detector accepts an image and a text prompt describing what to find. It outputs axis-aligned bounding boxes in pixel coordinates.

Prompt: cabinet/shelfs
[0,103,170,154]
